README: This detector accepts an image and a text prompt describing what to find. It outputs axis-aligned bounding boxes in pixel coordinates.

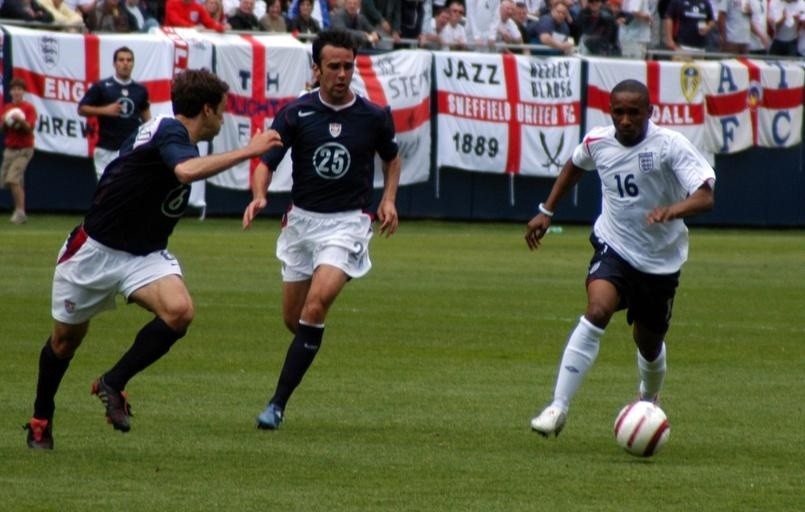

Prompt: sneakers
[256,402,284,428]
[9,208,30,226]
[89,374,133,432]
[23,418,54,451]
[530,405,567,436]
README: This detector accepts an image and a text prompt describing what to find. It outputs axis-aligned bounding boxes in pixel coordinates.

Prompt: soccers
[614,401,670,457]
[4,107,26,129]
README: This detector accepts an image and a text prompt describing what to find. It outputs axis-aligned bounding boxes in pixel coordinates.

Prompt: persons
[524,79,716,439]
[0,79,38,223]
[77,46,152,185]
[241,28,400,434]
[2,0,804,56]
[25,69,283,451]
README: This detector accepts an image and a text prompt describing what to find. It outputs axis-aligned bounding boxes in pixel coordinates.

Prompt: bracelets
[538,204,554,217]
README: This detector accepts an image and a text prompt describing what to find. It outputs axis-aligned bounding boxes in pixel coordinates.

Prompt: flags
[50,30,164,157]
[428,49,582,177]
[301,45,433,187]
[698,58,805,152]
[161,27,302,192]
[582,57,720,169]
